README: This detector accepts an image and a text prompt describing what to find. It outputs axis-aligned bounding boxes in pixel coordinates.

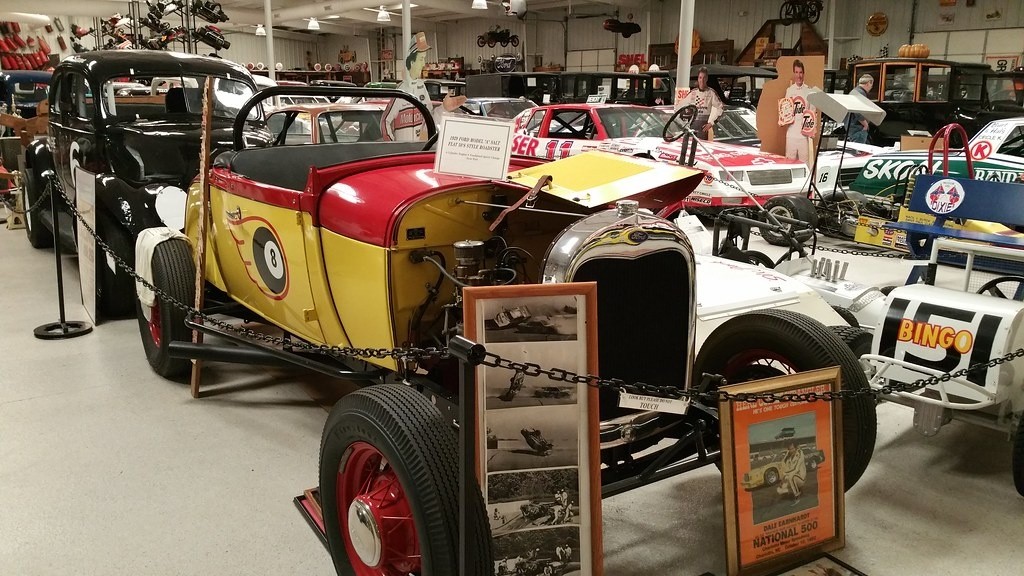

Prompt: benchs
[228,142,426,192]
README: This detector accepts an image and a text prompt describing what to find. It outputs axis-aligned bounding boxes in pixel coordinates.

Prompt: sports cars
[740,443,825,492]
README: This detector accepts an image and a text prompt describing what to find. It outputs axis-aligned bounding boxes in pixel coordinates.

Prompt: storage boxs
[900,135,943,151]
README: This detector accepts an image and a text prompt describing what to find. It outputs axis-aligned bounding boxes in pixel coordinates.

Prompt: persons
[777,440,807,499]
[785,58,816,167]
[498,544,572,576]
[494,489,574,525]
[380,31,466,141]
[843,74,874,144]
[675,66,723,139]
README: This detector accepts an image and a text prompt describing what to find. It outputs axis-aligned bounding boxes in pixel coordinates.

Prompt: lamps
[471,0,488,10]
[256,24,266,36]
[307,17,320,30]
[377,5,391,22]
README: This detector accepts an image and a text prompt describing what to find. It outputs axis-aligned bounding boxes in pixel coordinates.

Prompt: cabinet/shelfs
[255,71,370,87]
[647,40,734,69]
[422,70,476,82]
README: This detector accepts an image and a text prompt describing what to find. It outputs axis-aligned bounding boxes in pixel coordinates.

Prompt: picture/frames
[460,282,604,576]
[718,365,846,576]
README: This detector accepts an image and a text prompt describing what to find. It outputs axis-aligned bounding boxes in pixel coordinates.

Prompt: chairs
[165,88,224,116]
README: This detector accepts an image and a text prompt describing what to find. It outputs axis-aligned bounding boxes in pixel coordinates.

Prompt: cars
[509,104,817,227]
[0,70,56,205]
[129,84,876,576]
[21,49,278,379]
[849,116,1024,210]
